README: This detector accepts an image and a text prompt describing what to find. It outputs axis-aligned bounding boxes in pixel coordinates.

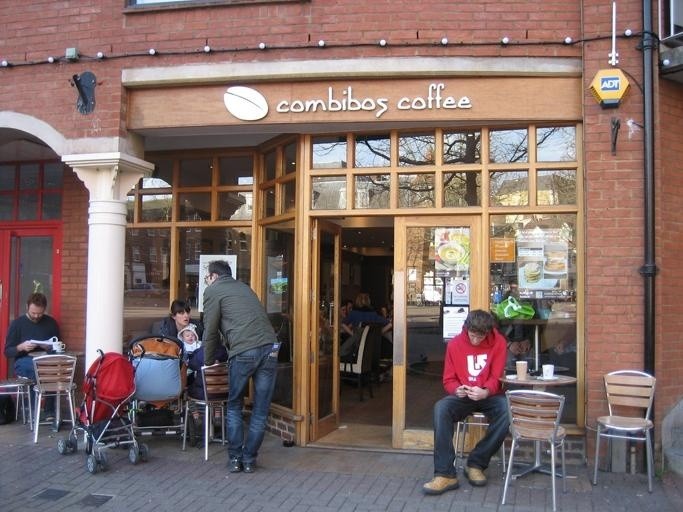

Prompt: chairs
[32,354,77,445]
[501,389,567,512]
[593,370,656,494]
[453,412,506,473]
[201,363,229,461]
[340,324,379,400]
[0,378,32,433]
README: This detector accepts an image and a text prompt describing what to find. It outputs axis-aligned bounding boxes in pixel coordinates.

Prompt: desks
[29,350,84,358]
[500,373,578,480]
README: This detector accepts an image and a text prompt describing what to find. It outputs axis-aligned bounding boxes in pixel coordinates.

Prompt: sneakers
[228,459,256,473]
[423,476,459,494]
[193,416,221,427]
[464,466,488,486]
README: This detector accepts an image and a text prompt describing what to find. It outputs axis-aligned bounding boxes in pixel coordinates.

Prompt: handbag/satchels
[0,395,14,425]
[491,295,535,320]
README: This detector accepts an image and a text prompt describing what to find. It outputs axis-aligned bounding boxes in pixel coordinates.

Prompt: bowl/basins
[437,243,465,265]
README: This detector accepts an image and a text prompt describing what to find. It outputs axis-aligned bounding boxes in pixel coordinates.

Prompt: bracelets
[506,340,513,349]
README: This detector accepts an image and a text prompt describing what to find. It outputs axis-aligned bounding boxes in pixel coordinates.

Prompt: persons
[498,272,540,390]
[319,292,393,383]
[4,292,61,423]
[202,259,281,474]
[159,298,203,343]
[186,331,229,430]
[422,309,507,495]
[177,323,202,379]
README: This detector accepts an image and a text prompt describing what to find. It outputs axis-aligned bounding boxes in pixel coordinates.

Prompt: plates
[536,376,559,381]
[543,258,567,276]
[518,266,558,289]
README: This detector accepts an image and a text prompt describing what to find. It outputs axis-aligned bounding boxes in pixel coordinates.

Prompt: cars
[124,282,162,292]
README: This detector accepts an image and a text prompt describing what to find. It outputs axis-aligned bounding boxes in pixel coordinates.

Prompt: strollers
[128,335,195,445]
[57,345,148,474]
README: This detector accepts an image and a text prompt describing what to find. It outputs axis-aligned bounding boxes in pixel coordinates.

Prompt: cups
[542,364,554,378]
[53,342,65,352]
[516,361,528,380]
